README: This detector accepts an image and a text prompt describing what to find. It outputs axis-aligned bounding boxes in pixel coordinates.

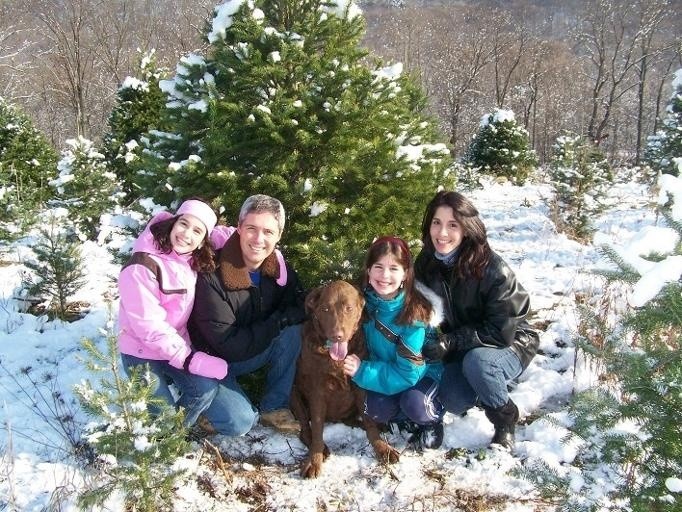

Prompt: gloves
[274,250,287,286]
[421,333,453,363]
[186,351,228,380]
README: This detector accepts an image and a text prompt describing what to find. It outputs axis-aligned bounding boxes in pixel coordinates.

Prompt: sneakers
[197,415,217,434]
[259,408,302,434]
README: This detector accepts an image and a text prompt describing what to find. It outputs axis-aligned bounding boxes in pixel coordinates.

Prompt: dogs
[290,279,400,480]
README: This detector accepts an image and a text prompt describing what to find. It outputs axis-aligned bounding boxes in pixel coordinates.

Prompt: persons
[415,190,541,454]
[188,194,304,435]
[117,197,288,435]
[344,235,448,451]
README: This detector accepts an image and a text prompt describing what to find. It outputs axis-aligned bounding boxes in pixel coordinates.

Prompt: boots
[420,422,443,449]
[484,399,519,453]
